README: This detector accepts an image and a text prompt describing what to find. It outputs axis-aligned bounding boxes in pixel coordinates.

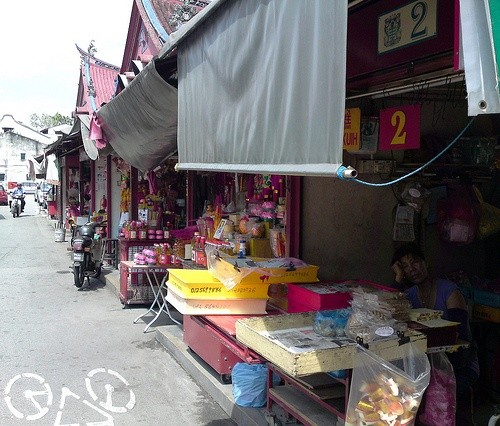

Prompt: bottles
[154,243,180,264]
[192,232,206,267]
[239,240,247,258]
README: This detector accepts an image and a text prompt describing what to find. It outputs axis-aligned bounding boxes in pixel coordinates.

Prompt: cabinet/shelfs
[265,362,351,426]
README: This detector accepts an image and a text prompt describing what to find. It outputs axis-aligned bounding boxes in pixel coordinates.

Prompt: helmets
[17,184,22,186]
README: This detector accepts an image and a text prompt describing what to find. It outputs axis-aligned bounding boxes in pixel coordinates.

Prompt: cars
[34,180,51,206]
[12,182,39,195]
[0,185,8,206]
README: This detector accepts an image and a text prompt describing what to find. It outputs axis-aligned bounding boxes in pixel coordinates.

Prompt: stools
[100,238,118,269]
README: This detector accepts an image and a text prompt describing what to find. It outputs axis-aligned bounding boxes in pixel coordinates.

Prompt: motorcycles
[69,209,108,287]
[10,191,26,218]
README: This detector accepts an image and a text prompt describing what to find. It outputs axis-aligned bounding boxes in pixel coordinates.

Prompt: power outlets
[356,159,374,173]
[385,160,397,174]
[373,160,385,174]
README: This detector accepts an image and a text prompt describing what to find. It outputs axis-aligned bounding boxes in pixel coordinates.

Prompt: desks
[117,235,173,262]
[119,259,184,333]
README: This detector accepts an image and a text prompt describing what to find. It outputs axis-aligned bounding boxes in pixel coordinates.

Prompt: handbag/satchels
[469,185,500,241]
[425,350,458,426]
[433,181,480,244]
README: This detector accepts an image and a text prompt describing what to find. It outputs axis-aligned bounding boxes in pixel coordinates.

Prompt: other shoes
[21,209,24,213]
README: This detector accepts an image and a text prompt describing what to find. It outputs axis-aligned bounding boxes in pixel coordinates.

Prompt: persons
[9,182,26,212]
[391,240,472,381]
